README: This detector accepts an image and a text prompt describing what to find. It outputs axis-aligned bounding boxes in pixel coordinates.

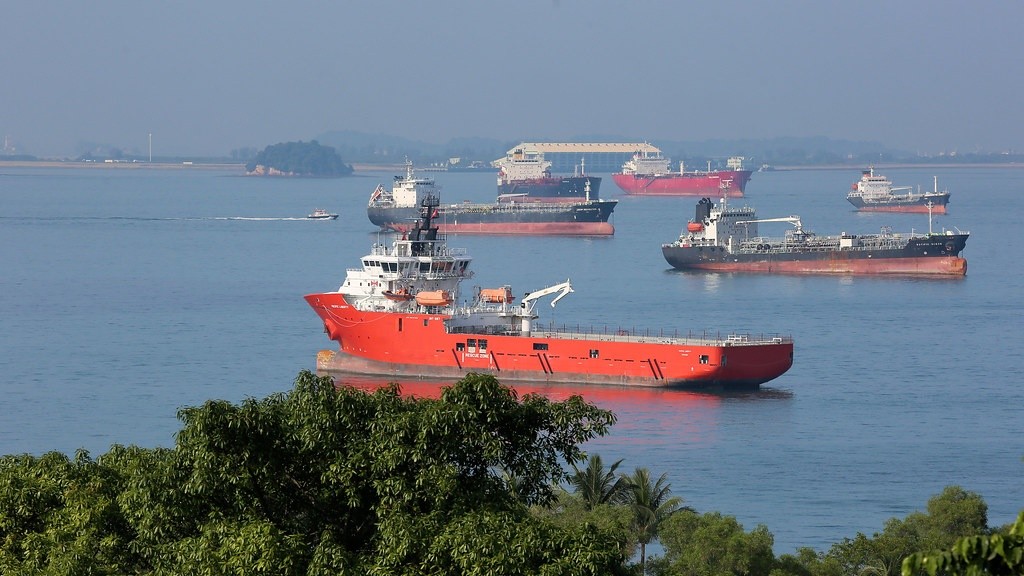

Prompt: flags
[430,209,438,219]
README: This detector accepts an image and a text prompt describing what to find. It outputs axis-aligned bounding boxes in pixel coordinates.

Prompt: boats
[847,164,951,214]
[307,208,339,220]
[611,145,756,198]
[305,193,797,391]
[367,155,618,238]
[497,154,601,200]
[659,173,973,276]
